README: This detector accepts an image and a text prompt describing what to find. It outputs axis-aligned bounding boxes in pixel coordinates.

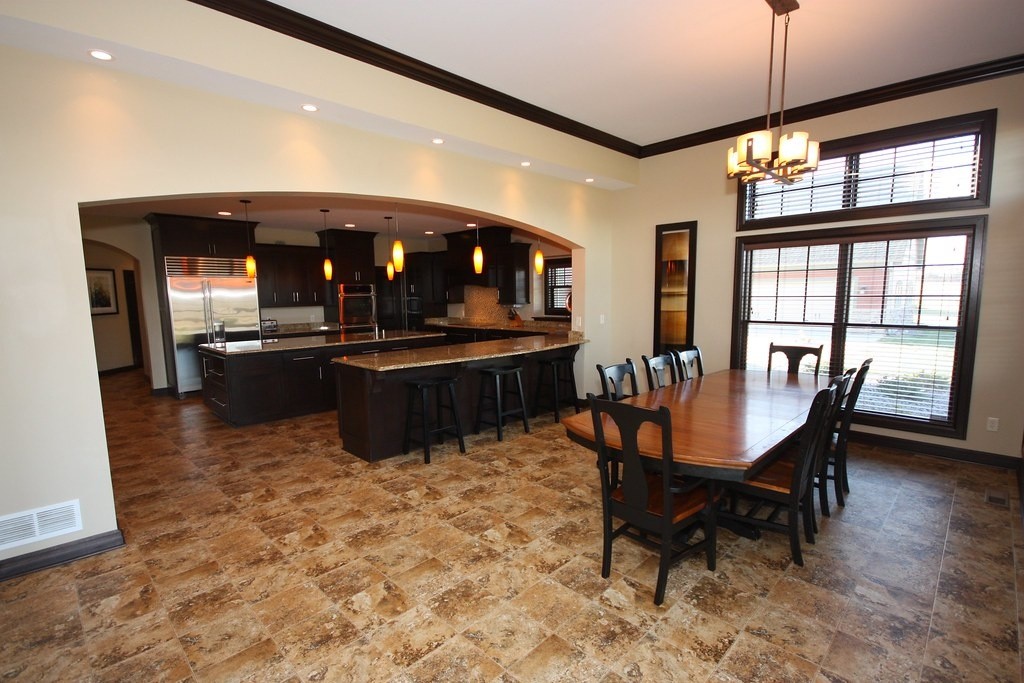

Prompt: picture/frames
[85,268,120,316]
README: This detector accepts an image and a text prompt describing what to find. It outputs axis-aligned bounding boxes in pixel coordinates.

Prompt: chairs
[720,357,873,568]
[674,345,704,382]
[596,357,639,485]
[766,341,824,374]
[641,350,677,391]
[585,392,727,607]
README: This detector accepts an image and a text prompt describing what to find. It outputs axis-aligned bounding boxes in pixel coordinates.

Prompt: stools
[402,375,465,464]
[529,356,580,423]
[473,366,529,442]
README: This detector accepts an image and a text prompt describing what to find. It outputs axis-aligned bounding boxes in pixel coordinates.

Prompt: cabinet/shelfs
[424,317,570,345]
[255,225,514,332]
[198,329,445,428]
[330,334,590,463]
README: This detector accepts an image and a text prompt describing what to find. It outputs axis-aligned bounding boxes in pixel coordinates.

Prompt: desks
[562,367,836,541]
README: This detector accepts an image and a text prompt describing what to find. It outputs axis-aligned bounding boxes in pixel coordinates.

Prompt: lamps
[726,0,821,186]
[392,202,404,272]
[319,209,333,280]
[535,236,544,275]
[473,218,483,274]
[384,216,394,281]
[239,200,257,279]
